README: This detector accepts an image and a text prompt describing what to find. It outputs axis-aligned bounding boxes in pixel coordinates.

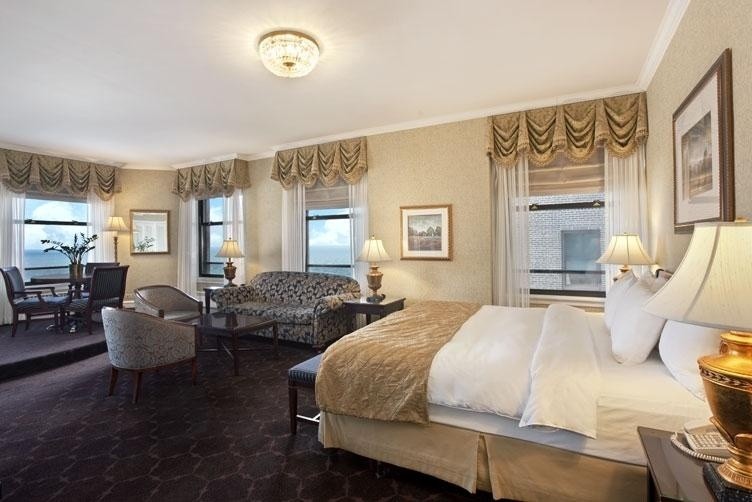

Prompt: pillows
[603,267,727,405]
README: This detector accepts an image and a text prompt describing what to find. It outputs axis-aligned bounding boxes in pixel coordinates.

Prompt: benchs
[285,349,326,437]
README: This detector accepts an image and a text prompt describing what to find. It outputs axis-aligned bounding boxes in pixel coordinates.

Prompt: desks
[338,293,407,338]
[30,274,93,334]
[201,281,234,314]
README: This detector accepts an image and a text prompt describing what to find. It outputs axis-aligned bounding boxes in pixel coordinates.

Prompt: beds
[311,264,676,501]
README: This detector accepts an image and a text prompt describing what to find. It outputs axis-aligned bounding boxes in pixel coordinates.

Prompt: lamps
[255,27,323,83]
[635,214,751,494]
[593,229,652,289]
[214,236,245,288]
[101,216,132,264]
[356,233,392,303]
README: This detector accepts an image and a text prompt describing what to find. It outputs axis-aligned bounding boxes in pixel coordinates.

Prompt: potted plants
[39,228,102,280]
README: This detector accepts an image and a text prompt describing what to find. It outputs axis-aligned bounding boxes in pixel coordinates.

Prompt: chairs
[0,264,73,336]
[64,262,123,329]
[132,281,203,325]
[95,303,200,409]
[62,264,132,336]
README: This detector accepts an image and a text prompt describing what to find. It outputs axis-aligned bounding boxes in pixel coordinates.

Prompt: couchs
[213,269,360,356]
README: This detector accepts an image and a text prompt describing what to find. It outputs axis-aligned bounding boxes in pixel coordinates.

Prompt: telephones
[682,419,734,457]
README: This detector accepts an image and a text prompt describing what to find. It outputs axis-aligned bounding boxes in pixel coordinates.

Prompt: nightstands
[632,425,719,501]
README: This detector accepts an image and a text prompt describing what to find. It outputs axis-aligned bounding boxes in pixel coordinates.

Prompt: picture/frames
[128,209,171,256]
[397,201,455,264]
[668,47,739,239]
